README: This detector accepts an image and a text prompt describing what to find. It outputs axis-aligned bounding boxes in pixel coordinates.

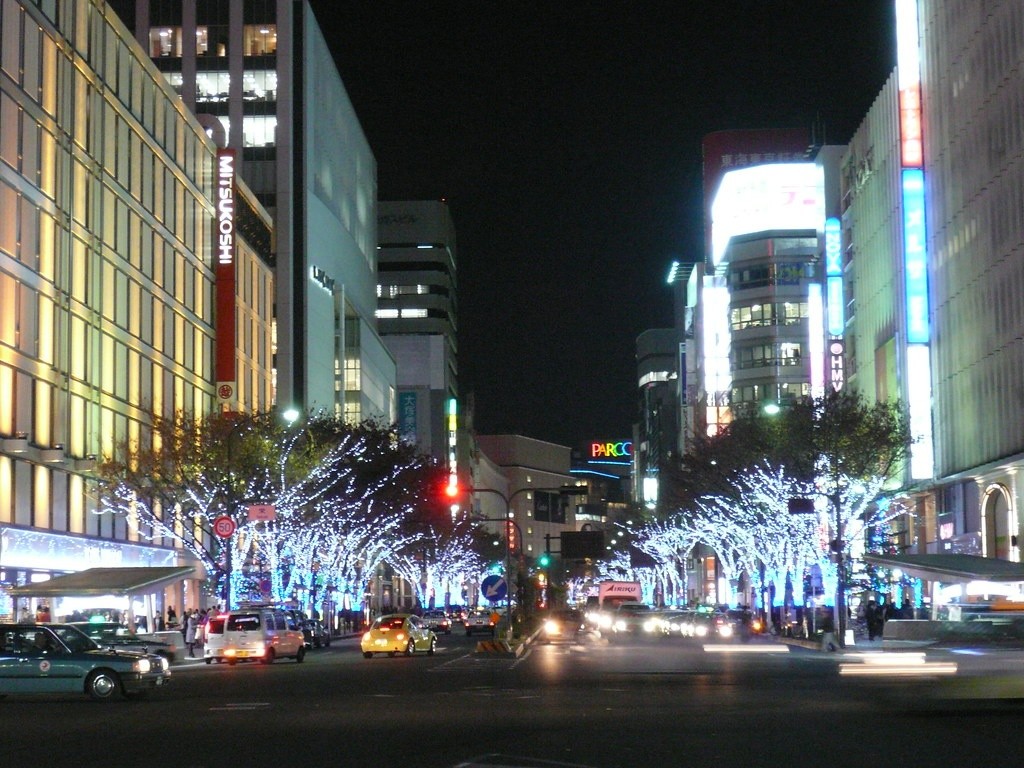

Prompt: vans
[222,609,308,666]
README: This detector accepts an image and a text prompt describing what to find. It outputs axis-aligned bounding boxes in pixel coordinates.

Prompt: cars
[66,619,176,670]
[545,578,762,646]
[423,611,452,635]
[291,610,332,651]
[1,622,173,707]
[203,615,228,663]
[447,604,508,623]
[361,614,438,657]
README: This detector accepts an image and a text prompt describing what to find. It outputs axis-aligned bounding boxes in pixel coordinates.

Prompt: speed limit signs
[214,516,236,539]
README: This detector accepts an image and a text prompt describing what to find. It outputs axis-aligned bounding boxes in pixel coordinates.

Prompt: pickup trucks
[464,607,496,638]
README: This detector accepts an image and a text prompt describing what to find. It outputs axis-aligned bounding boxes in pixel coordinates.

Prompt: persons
[154,605,223,657]
[19,604,50,623]
[65,608,129,628]
[819,597,928,640]
